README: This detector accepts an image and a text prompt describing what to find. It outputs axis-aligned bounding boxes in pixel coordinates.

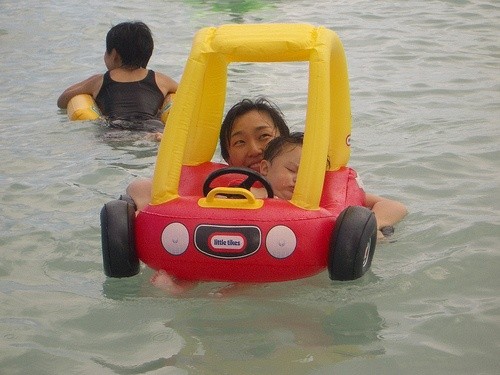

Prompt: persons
[152,128,331,302]
[57,21,178,142]
[124,95,409,239]
